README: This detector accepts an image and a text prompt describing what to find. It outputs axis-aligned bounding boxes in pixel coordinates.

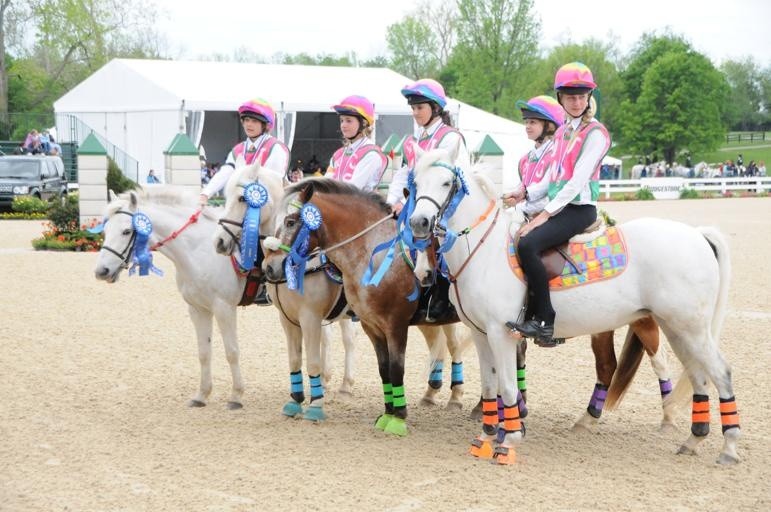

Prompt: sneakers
[424,287,451,319]
[253,285,269,304]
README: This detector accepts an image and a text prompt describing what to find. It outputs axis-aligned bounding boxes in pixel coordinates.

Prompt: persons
[517,95,566,348]
[600,154,771,194]
[195,98,294,306]
[384,79,475,325]
[323,96,388,317]
[494,61,612,341]
[201,163,220,183]
[24,129,61,156]
[147,169,158,183]
[288,155,327,183]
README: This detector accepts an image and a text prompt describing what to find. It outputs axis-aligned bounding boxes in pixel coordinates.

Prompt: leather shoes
[504,321,555,343]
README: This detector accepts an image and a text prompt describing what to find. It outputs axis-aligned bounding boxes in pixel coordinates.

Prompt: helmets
[400,78,447,111]
[332,94,377,129]
[515,93,565,129]
[552,62,597,94]
[237,97,276,132]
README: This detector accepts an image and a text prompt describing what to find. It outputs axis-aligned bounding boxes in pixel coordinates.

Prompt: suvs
[1,156,70,212]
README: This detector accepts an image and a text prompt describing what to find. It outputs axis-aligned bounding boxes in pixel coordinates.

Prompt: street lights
[3,73,21,118]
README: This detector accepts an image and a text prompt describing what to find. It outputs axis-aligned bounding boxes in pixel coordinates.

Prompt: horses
[630,162,666,179]
[211,152,467,423]
[259,173,529,438]
[400,185,681,438]
[405,138,742,467]
[92,182,357,413]
[674,160,708,179]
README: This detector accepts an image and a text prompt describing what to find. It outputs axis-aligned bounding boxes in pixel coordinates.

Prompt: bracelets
[523,183,531,201]
